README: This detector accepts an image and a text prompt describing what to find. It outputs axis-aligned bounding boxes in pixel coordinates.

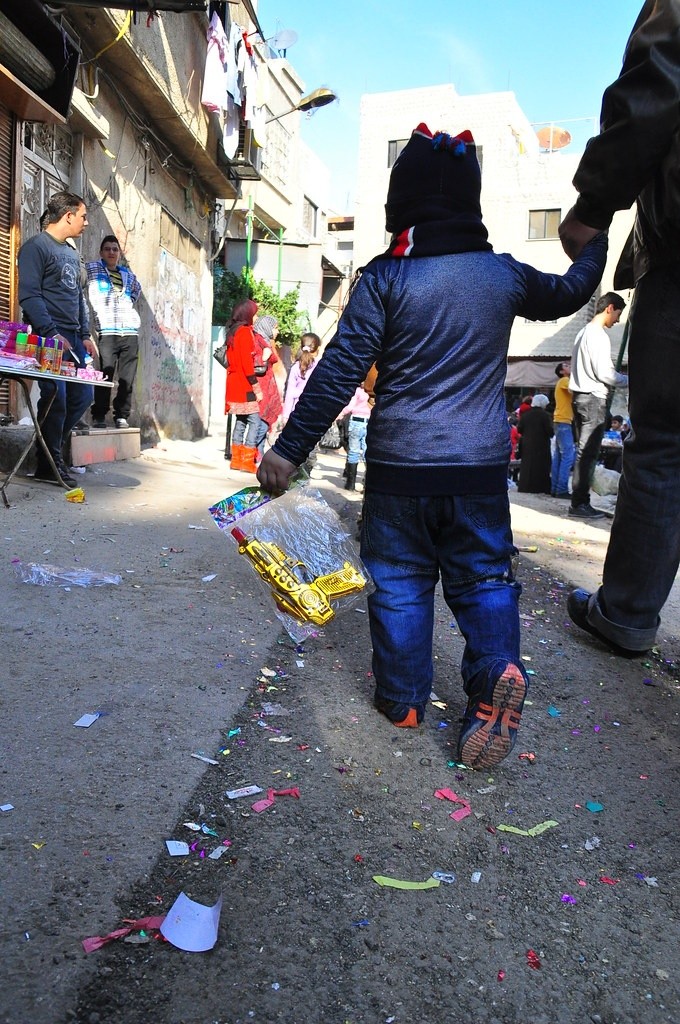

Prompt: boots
[230,444,258,473]
[344,462,357,490]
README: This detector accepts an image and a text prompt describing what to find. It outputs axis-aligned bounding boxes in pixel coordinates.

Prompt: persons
[507,292,629,518]
[566,0,680,660]
[257,123,610,772]
[226,298,374,491]
[17,192,141,487]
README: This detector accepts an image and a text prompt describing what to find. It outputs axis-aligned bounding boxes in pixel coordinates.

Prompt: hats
[385,121,481,232]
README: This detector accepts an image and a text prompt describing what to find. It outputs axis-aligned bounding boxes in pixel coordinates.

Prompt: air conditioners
[218,112,262,178]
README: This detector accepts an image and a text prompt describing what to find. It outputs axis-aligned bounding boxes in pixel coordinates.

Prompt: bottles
[15,332,63,374]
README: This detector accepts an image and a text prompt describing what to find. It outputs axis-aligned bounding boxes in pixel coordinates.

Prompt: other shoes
[551,491,573,499]
[92,418,106,428]
[456,658,530,769]
[115,418,129,428]
[567,589,649,657]
[253,449,264,462]
[72,418,89,430]
[373,682,426,727]
[57,446,70,472]
[568,503,605,518]
[33,460,77,488]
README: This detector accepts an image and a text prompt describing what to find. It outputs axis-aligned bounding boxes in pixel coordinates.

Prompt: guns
[231,526,370,626]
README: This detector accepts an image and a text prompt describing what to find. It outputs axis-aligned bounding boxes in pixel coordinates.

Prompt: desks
[0,366,114,507]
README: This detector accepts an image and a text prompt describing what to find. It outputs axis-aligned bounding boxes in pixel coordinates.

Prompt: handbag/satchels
[213,333,267,376]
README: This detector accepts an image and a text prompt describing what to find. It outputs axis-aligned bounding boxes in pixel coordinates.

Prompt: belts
[352,417,369,423]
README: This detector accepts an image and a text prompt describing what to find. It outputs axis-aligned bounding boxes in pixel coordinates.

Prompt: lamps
[267,89,336,124]
